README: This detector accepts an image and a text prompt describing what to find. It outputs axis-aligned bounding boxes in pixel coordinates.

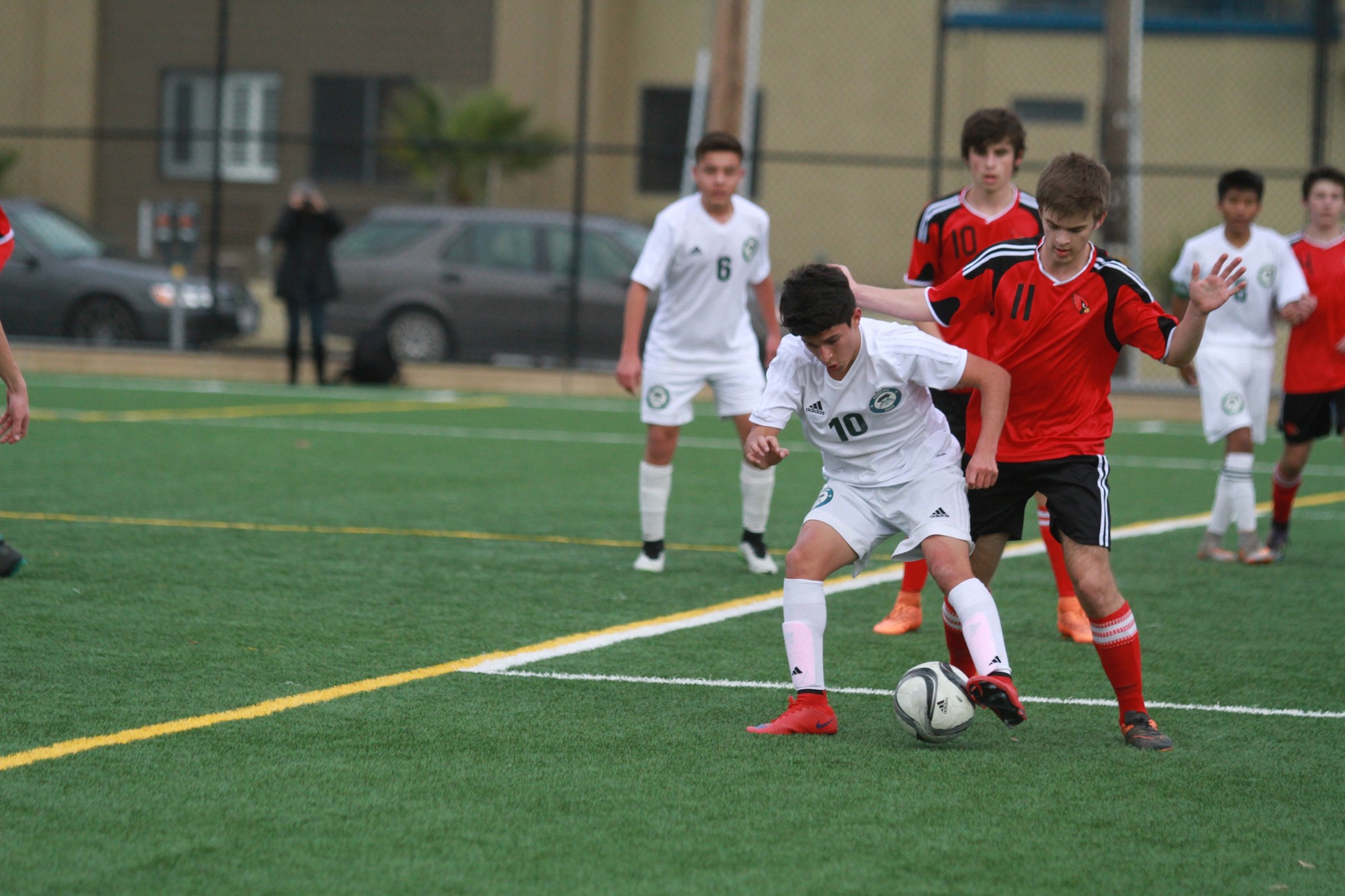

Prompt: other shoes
[1238,545,1272,564]
[1267,527,1290,563]
[1197,546,1235,563]
[0,542,22,578]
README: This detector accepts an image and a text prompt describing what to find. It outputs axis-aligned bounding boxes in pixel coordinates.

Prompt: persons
[0,208,28,579]
[742,262,1026,736]
[823,151,1247,751]
[1265,167,1345,560]
[615,130,782,575]
[872,107,1094,642]
[272,174,343,385]
[1169,167,1315,563]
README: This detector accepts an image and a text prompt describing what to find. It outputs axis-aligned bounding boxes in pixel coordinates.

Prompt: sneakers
[1121,709,1174,752]
[874,603,923,635]
[746,693,836,736]
[1056,605,1093,644]
[965,674,1028,728]
[634,545,665,573]
[738,537,777,575]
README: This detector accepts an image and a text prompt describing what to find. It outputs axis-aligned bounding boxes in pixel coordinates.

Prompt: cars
[327,206,792,366]
[1,195,254,348]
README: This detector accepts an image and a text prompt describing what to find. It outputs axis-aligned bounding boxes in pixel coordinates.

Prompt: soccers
[891,660,978,744]
